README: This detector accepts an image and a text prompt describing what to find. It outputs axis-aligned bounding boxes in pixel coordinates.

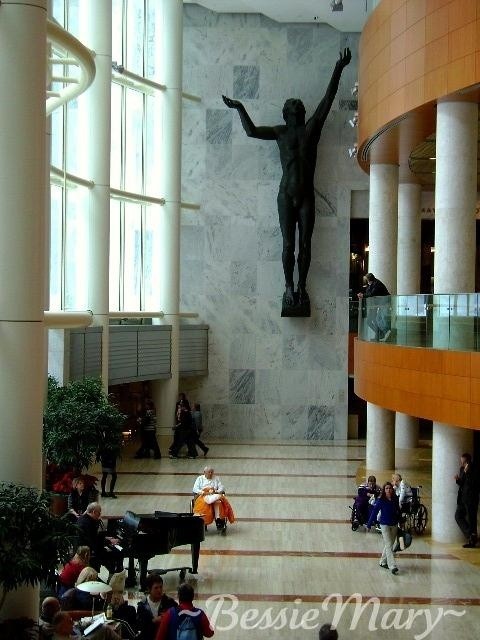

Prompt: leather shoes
[469,535,477,545]
[463,544,475,547]
[204,448,209,456]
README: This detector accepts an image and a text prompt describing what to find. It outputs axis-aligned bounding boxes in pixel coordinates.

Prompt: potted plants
[42,372,128,514]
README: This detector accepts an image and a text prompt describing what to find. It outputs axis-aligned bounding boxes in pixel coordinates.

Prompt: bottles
[107,604,113,620]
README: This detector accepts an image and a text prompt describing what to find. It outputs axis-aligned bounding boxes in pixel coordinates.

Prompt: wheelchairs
[400,484,429,535]
[189,490,229,534]
[348,505,381,533]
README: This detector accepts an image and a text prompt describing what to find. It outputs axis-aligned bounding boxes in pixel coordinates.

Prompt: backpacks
[168,605,202,640]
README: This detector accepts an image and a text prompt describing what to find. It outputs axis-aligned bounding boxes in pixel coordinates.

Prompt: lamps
[346,82,357,160]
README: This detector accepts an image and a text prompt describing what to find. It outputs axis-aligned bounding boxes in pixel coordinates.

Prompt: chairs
[37,539,132,640]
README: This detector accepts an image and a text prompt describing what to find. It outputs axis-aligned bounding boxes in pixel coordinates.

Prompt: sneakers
[188,457,195,459]
[379,563,389,569]
[169,456,176,459]
[392,567,398,574]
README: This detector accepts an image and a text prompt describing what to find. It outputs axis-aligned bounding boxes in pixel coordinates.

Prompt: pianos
[102,511,205,592]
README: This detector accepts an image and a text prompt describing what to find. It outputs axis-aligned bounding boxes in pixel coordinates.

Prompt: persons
[363,275,384,340]
[453,452,479,547]
[357,272,393,342]
[365,481,404,575]
[354,475,382,524]
[37,390,236,639]
[391,473,414,528]
[317,623,339,640]
[216,44,355,317]
